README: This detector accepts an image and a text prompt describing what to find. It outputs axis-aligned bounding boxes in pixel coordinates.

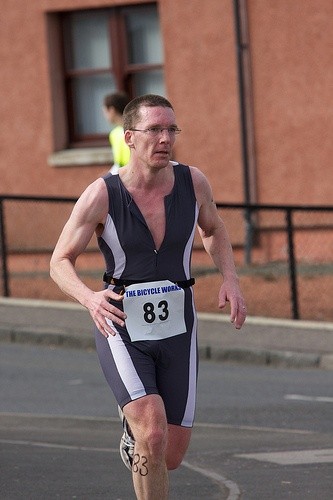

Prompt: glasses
[129,128,181,134]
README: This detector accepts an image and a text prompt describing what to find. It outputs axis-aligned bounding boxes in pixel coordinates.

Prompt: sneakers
[118,404,136,471]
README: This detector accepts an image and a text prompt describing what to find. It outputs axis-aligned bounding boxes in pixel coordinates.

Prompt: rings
[242,305,247,311]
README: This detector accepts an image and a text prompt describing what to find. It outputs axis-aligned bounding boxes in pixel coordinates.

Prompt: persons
[49,94,249,500]
[100,89,134,175]
